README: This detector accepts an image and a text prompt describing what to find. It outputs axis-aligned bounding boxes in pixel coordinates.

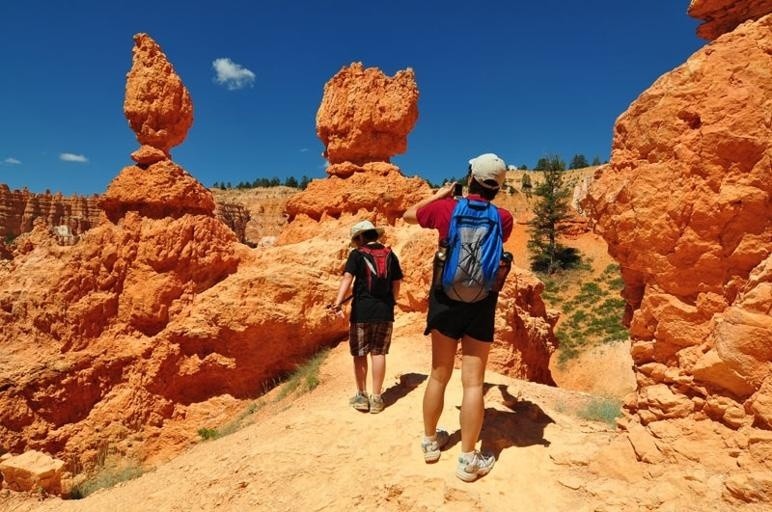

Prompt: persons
[333,220,403,415]
[403,153,513,482]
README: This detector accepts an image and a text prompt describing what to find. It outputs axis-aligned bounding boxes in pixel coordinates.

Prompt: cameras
[455,184,462,195]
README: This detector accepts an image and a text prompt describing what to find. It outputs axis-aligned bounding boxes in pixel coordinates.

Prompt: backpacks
[359,246,392,301]
[434,196,512,303]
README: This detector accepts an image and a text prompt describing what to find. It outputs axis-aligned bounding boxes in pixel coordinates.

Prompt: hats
[469,153,506,190]
[351,220,385,243]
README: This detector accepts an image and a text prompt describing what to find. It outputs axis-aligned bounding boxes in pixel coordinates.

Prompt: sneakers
[350,394,386,413]
[455,451,495,481]
[423,429,449,462]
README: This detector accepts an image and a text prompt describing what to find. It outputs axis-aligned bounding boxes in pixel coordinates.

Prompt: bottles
[434,240,448,286]
[493,252,513,293]
